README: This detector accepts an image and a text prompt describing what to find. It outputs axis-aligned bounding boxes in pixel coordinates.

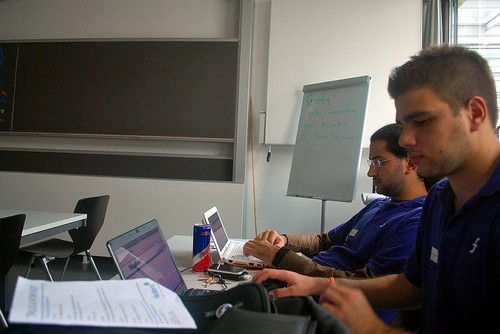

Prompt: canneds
[192,223,211,272]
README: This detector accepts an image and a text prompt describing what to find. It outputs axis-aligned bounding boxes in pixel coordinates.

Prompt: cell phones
[207,264,249,281]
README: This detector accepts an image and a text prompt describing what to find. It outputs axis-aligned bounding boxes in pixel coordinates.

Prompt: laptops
[203,205,271,267]
[107,220,223,295]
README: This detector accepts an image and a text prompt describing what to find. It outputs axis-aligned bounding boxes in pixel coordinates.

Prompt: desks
[110,234,303,292]
[0,209,87,248]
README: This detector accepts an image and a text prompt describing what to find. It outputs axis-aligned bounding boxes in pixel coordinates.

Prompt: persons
[244,123,429,280]
[252,44,500,334]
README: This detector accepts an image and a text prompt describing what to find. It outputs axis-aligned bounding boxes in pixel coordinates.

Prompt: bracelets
[272,246,289,267]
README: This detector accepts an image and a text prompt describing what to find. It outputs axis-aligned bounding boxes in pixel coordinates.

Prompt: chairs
[21,195,110,282]
[0,214,26,331]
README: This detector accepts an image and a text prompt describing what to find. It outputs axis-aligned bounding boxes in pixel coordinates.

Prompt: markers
[295,193,314,199]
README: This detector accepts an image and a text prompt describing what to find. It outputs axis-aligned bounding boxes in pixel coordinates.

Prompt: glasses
[367,157,396,168]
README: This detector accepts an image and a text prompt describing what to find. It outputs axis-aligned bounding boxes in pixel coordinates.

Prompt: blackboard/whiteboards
[0,38,252,185]
[284,75,372,202]
